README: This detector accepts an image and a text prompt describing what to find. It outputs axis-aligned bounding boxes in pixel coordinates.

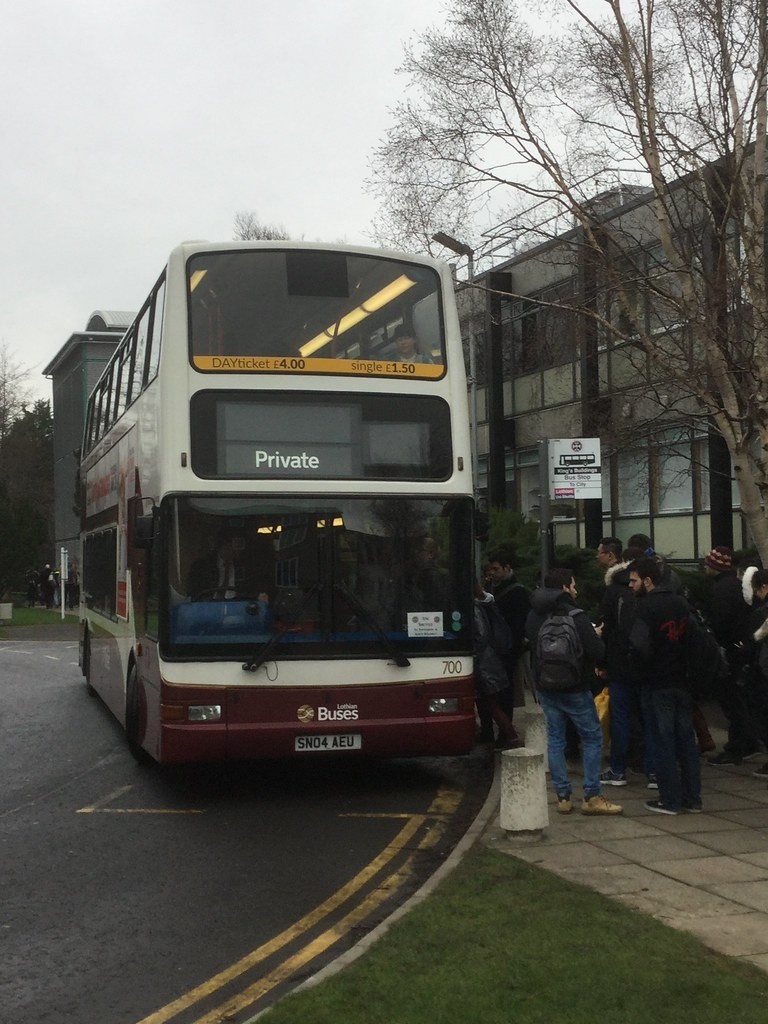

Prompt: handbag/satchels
[686,611,729,680]
[48,571,56,587]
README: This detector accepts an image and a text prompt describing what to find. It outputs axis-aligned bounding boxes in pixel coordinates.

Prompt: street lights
[437,231,483,596]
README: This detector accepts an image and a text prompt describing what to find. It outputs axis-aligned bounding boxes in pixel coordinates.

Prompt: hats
[703,546,733,572]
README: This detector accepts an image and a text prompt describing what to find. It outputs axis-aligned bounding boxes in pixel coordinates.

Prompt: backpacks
[533,608,594,692]
[474,602,516,667]
[613,587,637,655]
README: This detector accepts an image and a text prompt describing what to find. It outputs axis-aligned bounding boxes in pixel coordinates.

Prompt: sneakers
[598,770,627,786]
[581,794,623,815]
[644,800,678,815]
[556,792,574,814]
[681,798,703,813]
[647,773,659,789]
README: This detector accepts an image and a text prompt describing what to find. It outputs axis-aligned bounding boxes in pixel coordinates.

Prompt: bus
[73,236,494,781]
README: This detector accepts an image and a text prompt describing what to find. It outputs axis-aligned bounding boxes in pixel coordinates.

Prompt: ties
[214,562,231,601]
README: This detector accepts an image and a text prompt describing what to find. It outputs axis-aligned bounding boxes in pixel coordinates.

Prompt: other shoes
[478,733,494,744]
[494,736,510,752]
[508,733,519,746]
[707,751,743,766]
[742,742,762,760]
[753,762,768,777]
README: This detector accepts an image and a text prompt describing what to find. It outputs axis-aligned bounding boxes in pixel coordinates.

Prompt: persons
[387,324,435,364]
[474,532,768,817]
[410,535,445,614]
[190,526,276,604]
[25,563,80,611]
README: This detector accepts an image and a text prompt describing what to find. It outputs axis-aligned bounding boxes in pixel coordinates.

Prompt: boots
[693,710,715,755]
